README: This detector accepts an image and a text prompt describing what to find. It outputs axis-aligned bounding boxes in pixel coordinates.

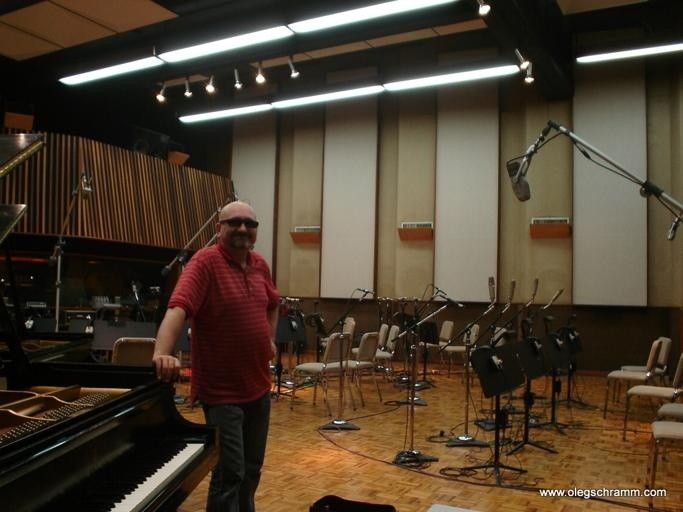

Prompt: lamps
[155,55,300,104]
[476,1,493,18]
[515,48,536,85]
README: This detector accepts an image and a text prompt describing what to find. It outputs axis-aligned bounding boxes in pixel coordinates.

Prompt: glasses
[218,216,260,229]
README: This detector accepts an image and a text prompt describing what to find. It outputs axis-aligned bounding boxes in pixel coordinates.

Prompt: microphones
[80,171,93,200]
[286,297,304,304]
[506,162,530,201]
[357,277,564,308]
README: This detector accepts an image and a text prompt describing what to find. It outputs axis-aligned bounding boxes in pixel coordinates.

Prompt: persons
[145,197,281,511]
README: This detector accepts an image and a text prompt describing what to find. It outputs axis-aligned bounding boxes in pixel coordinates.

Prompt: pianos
[1,360,219,512]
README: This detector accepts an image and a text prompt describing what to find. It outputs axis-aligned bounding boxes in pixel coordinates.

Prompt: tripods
[555,362,588,406]
[506,378,559,454]
[528,364,568,435]
[461,394,527,486]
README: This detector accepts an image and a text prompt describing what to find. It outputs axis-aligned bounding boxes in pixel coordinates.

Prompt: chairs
[418,320,507,388]
[600,335,683,512]
[111,335,157,364]
[284,317,402,420]
